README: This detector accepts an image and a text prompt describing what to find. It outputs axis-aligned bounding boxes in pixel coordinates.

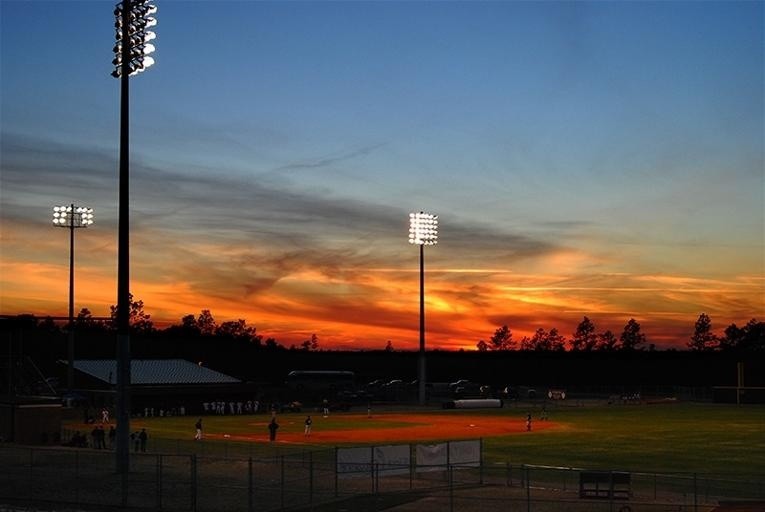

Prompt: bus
[285,369,355,390]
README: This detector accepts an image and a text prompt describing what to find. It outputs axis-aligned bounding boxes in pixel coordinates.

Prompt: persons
[322,398,330,417]
[305,416,312,438]
[101,407,110,424]
[268,418,279,442]
[194,418,203,441]
[50,424,147,455]
[620,391,642,406]
[138,400,281,416]
[526,413,532,430]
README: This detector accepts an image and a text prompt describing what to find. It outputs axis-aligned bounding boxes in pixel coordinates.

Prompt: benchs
[579,471,631,500]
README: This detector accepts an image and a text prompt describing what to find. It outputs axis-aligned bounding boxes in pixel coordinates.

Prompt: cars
[449,380,566,399]
[361,376,433,396]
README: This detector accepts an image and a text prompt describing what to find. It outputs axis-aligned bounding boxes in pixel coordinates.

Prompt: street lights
[409,212,438,406]
[113,0,158,490]
[53,204,93,319]
